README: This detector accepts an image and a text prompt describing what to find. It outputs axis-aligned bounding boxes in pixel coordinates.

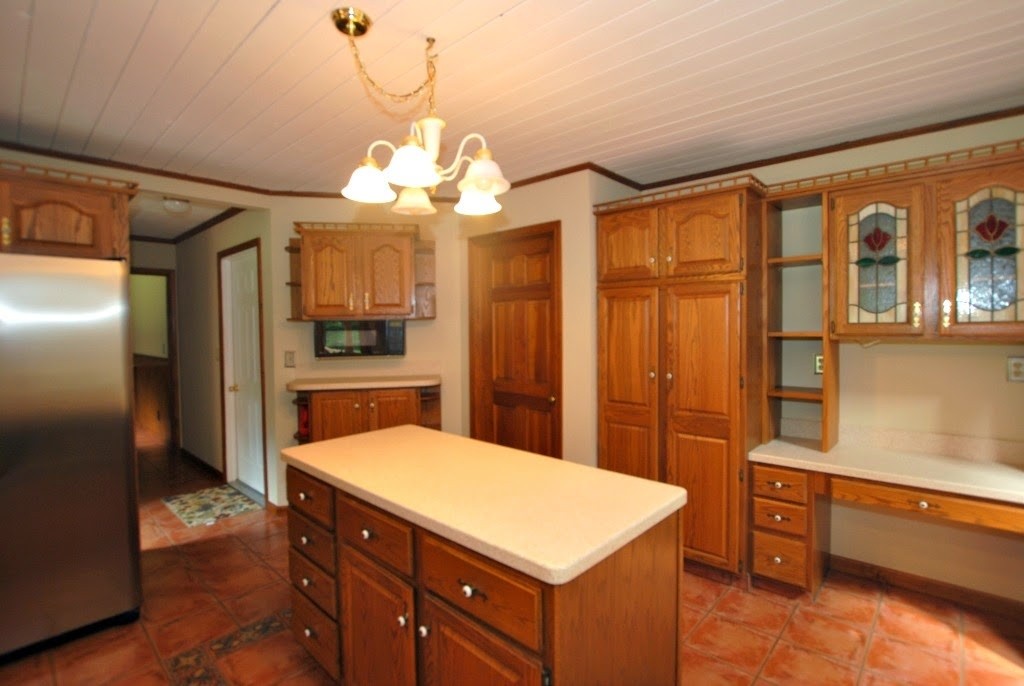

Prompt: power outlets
[1007,356,1024,381]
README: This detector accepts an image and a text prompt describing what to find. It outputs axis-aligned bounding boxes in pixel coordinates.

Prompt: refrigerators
[0,253,145,655]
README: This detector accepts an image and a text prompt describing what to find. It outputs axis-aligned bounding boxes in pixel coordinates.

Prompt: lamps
[331,7,510,216]
[163,196,191,213]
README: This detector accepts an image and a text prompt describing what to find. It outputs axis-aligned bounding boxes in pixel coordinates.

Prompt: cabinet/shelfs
[337,543,545,686]
[830,160,1024,346]
[760,189,838,451]
[0,175,114,260]
[597,281,728,564]
[311,388,419,443]
[595,189,742,284]
[293,221,421,320]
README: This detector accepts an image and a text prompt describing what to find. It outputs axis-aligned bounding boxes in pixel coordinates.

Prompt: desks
[747,432,1024,605]
[287,374,442,446]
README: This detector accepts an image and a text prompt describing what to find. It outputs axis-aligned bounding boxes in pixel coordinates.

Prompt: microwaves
[315,319,404,357]
